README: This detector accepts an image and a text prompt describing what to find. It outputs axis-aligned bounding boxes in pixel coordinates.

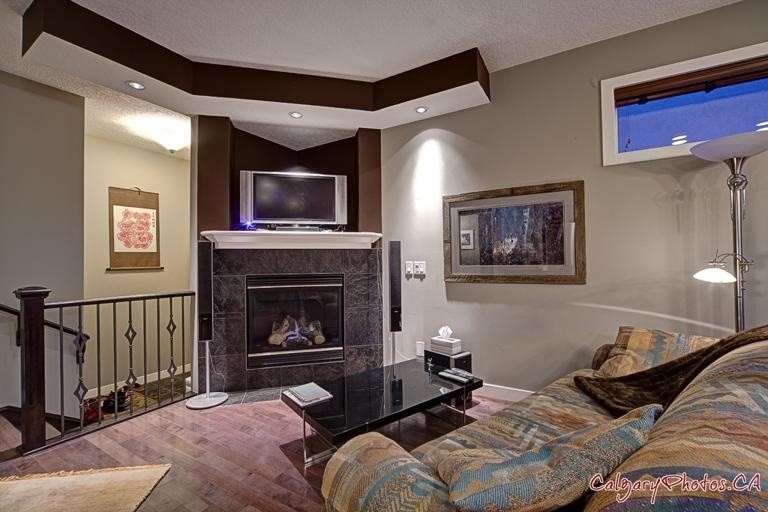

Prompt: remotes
[444,369,471,380]
[438,372,470,384]
[452,368,473,376]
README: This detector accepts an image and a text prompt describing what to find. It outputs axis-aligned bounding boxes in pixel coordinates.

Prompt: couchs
[320,342,768,512]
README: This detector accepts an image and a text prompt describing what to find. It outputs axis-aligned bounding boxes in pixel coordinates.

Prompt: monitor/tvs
[240,170,347,231]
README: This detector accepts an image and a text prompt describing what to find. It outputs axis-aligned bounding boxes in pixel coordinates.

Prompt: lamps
[689,131,767,333]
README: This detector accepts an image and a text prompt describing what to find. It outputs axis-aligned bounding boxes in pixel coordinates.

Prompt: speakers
[196,240,214,341]
[391,378,403,411]
[390,242,403,330]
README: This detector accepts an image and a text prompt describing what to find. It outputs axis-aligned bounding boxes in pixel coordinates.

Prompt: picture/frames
[442,179,587,286]
[601,40,768,167]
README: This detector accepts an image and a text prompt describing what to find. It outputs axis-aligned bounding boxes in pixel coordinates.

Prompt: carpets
[0,463,173,511]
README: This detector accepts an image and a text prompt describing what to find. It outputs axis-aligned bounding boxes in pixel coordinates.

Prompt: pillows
[594,325,722,378]
[585,340,768,512]
[438,403,664,510]
